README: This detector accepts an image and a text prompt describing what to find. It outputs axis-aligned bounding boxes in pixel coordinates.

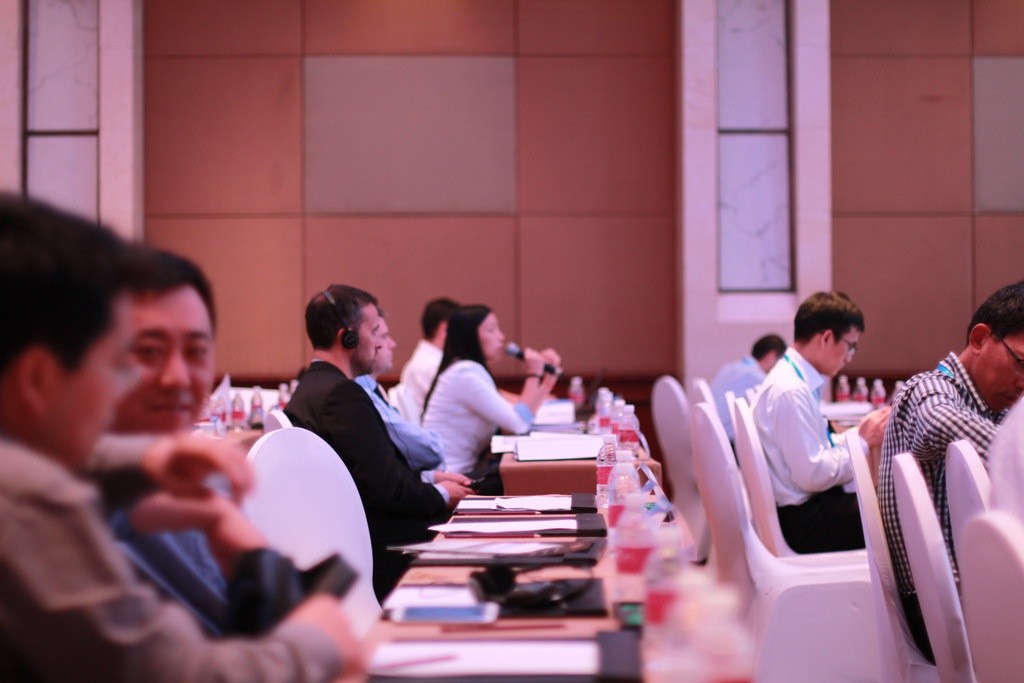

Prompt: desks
[499,414,663,495]
[355,494,740,683]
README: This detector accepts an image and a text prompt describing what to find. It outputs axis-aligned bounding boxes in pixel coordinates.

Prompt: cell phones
[390,603,499,624]
[467,478,487,490]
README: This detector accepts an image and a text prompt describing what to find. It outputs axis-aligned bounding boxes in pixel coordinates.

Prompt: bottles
[616,492,655,606]
[891,381,903,405]
[642,527,755,683]
[597,387,613,435]
[837,375,850,401]
[232,392,246,431]
[596,436,621,508]
[853,376,868,402]
[608,449,642,557]
[569,375,586,409]
[214,397,228,437]
[871,378,886,408]
[614,398,640,449]
[251,387,263,424]
[279,383,291,410]
[195,405,211,425]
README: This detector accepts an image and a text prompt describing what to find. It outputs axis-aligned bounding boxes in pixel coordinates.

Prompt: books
[512,438,605,461]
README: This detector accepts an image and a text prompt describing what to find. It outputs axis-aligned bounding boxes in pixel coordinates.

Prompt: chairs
[956,506,1024,683]
[945,439,991,554]
[893,451,977,683]
[844,427,940,683]
[242,425,383,619]
[690,376,720,576]
[730,396,867,621]
[651,373,712,566]
[690,402,880,683]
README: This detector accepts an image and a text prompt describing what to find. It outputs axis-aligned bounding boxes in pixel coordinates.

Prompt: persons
[0,189,364,683]
[399,297,458,426]
[422,306,560,492]
[749,291,892,555]
[284,284,478,602]
[876,284,1024,666]
[356,308,445,474]
[712,334,787,466]
[101,245,312,633]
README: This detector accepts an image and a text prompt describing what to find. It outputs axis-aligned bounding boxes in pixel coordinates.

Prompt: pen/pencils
[442,534,541,540]
[377,651,457,668]
[453,511,541,515]
[440,623,567,633]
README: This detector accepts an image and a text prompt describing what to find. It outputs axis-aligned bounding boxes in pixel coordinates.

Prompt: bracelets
[525,373,542,379]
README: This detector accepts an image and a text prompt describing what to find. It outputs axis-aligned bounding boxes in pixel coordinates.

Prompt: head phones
[226,547,358,638]
[468,560,595,612]
[323,290,359,349]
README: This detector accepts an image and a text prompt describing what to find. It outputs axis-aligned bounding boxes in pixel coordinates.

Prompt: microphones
[505,342,564,376]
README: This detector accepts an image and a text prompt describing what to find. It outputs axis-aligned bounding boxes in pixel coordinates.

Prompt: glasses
[833,331,858,354]
[994,332,1024,373]
